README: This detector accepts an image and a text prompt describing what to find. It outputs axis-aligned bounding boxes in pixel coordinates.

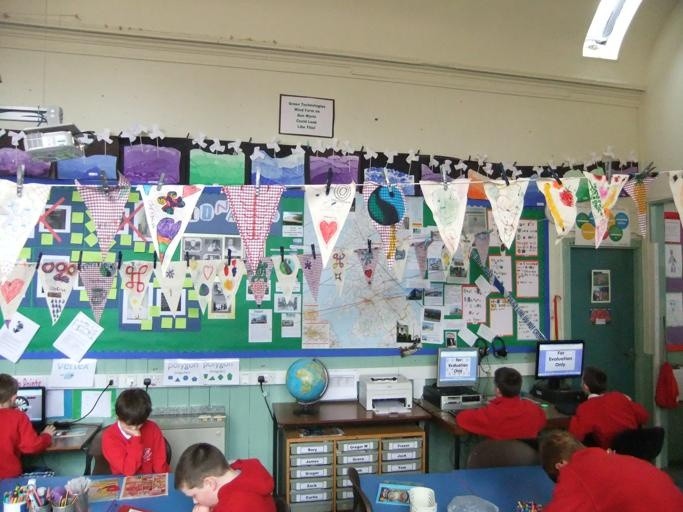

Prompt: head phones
[475,337,491,356]
[492,336,507,358]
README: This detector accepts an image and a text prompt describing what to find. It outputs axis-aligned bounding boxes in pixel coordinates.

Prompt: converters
[54,422,70,428]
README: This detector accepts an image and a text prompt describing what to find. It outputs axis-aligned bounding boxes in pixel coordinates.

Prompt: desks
[272,399,431,512]
[360,464,563,511]
[0,423,212,512]
[423,387,611,472]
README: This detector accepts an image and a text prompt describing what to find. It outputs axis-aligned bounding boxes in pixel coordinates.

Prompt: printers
[358,372,415,414]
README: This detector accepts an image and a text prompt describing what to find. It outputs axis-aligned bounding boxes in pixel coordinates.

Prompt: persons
[172,441,278,511]
[0,373,56,475]
[539,430,683,512]
[100,388,171,477]
[455,366,547,440]
[565,365,647,447]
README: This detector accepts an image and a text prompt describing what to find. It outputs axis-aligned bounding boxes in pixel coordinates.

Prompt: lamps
[595,0,629,65]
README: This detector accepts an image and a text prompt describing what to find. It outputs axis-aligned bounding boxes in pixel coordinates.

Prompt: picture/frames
[279,94,335,139]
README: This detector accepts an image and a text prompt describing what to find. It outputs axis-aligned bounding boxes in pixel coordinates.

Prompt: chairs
[344,467,372,512]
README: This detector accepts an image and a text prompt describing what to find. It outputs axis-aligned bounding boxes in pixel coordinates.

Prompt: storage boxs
[290,437,423,511]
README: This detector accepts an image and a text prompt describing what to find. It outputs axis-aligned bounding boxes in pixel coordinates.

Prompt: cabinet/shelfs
[282,423,426,512]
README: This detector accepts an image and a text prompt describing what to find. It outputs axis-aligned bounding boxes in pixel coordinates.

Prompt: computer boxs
[531,384,587,403]
[423,386,483,412]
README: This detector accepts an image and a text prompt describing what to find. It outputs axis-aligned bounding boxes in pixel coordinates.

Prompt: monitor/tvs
[536,340,586,390]
[436,348,480,393]
[15,386,45,431]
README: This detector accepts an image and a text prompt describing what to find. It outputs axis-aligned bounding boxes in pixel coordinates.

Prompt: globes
[286,358,329,414]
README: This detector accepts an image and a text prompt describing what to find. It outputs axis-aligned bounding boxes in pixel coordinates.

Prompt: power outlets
[104,374,161,388]
[256,373,272,385]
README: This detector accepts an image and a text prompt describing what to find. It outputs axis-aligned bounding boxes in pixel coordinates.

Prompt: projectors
[21,125,86,164]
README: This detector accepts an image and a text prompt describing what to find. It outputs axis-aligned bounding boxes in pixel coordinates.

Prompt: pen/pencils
[4,481,90,507]
